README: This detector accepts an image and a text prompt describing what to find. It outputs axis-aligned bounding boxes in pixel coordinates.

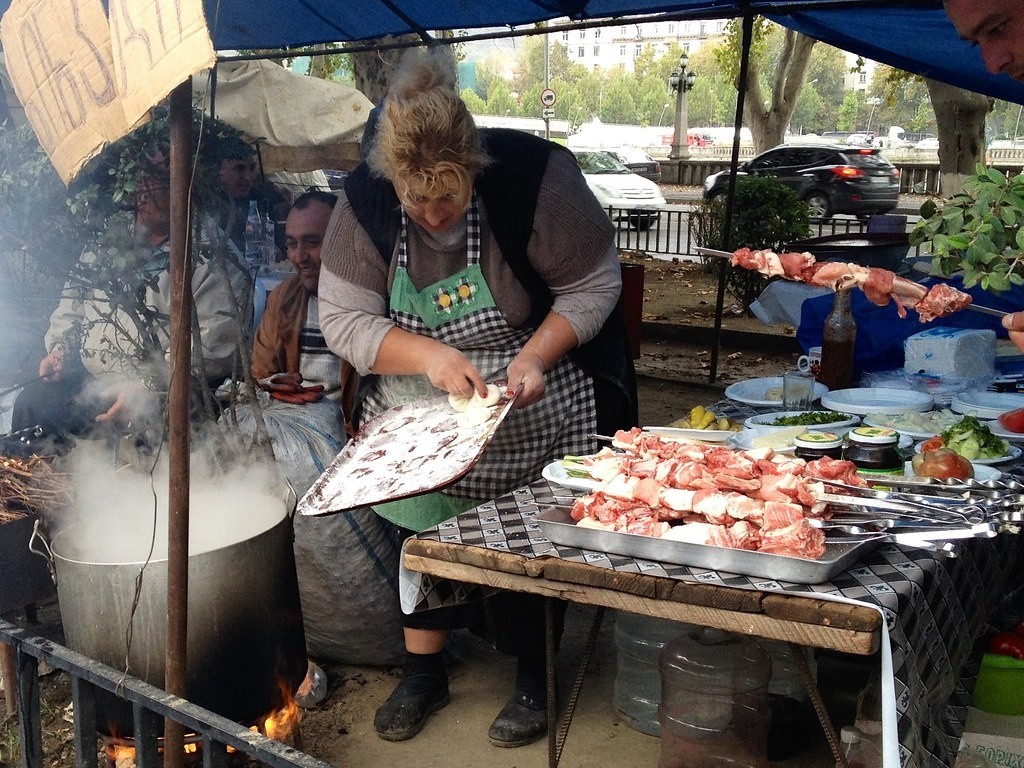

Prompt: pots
[28,477,309,745]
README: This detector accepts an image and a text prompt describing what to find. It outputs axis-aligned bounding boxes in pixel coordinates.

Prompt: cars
[598,147,662,184]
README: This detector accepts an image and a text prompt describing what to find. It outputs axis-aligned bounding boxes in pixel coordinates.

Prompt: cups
[782,371,816,411]
[798,346,822,377]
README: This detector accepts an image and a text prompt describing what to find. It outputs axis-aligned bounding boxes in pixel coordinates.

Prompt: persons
[1002,309,1024,357]
[13,135,374,435]
[318,47,623,747]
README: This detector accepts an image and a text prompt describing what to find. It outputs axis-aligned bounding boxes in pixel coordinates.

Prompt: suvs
[702,143,901,225]
[566,147,667,230]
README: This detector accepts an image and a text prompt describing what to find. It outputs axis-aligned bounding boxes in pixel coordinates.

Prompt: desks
[399,368,1024,768]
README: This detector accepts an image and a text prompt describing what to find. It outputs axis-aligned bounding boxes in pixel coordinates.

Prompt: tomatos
[998,407,1024,433]
[913,437,975,479]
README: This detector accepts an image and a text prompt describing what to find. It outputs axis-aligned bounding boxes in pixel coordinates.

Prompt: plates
[863,415,965,438]
[986,420,1024,438]
[905,460,1002,484]
[725,375,828,407]
[541,454,599,491]
[914,439,1022,464]
[641,425,736,449]
[820,388,934,414]
[731,429,823,451]
[951,392,1024,419]
[744,412,860,430]
[827,426,913,449]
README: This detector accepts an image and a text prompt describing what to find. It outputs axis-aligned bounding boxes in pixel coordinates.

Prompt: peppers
[690,405,716,430]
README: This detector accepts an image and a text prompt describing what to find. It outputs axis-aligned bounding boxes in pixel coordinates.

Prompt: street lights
[666,52,696,161]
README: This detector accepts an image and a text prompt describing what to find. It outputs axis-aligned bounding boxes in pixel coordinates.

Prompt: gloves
[214,371,325,405]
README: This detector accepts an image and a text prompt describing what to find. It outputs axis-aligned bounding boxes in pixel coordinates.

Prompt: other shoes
[374,664,450,741]
[488,672,562,748]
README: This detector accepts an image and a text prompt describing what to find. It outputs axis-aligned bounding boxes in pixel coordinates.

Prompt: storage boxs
[954,705,1024,768]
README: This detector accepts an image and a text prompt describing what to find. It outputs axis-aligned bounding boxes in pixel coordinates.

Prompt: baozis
[764,384,810,400]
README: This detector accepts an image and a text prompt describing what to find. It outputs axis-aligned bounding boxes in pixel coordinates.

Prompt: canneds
[793,427,904,494]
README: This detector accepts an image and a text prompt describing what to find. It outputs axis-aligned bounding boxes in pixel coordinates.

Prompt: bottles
[245,200,275,270]
[818,288,856,392]
[613,607,813,767]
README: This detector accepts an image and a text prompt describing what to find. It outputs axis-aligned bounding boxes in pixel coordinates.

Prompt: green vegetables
[758,411,853,425]
[941,414,1014,461]
